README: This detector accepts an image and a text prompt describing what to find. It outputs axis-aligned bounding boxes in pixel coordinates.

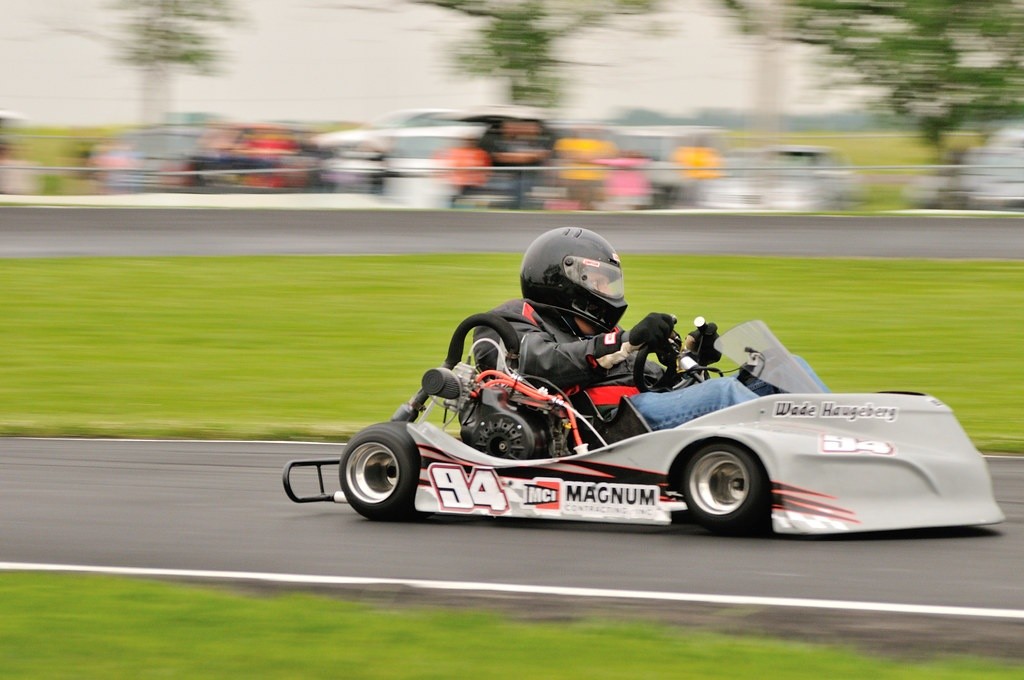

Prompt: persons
[474,227,835,433]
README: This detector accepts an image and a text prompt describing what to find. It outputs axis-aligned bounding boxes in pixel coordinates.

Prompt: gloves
[685,321,718,351]
[594,311,678,369]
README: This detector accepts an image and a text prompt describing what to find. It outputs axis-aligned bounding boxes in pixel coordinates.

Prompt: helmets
[520,226,629,337]
